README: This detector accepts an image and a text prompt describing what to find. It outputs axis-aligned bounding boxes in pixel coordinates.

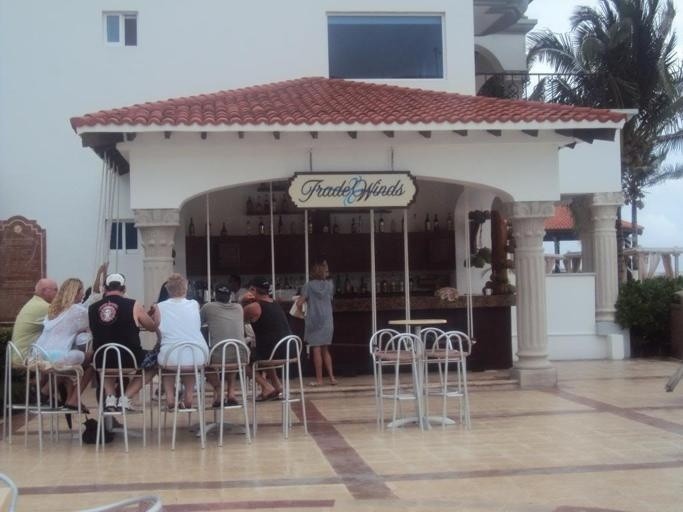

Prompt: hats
[105,273,125,290]
[215,281,231,294]
[253,278,269,289]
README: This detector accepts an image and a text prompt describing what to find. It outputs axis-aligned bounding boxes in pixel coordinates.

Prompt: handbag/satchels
[290,299,306,319]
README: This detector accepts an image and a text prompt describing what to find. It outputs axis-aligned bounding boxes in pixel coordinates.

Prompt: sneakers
[118,396,135,411]
[30,384,61,407]
[106,395,118,412]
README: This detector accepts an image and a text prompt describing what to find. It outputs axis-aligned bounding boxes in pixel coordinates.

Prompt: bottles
[188,213,454,237]
[188,272,414,304]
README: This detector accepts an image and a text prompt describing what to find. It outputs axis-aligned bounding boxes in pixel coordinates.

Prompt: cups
[486,288,493,295]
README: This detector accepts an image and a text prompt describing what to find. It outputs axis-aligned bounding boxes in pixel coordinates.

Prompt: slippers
[63,402,90,413]
[309,379,338,386]
[212,399,240,407]
[256,388,279,402]
[161,402,193,412]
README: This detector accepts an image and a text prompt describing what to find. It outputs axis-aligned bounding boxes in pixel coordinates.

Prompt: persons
[10,263,296,413]
[296,260,338,386]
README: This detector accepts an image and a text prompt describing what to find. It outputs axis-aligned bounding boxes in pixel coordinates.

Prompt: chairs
[368,327,472,432]
